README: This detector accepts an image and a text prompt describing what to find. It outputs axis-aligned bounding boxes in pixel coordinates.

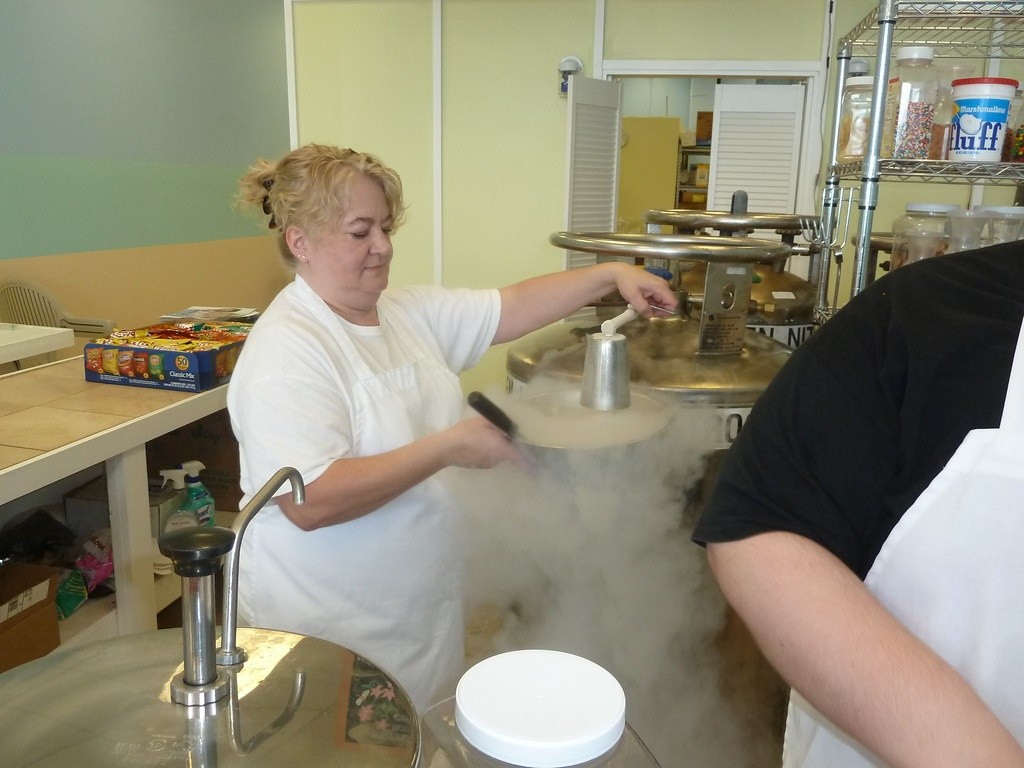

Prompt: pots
[494,389,674,479]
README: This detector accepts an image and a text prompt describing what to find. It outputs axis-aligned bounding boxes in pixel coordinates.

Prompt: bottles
[838,76,876,159]
[891,203,1024,272]
[880,45,940,161]
[947,77,1019,161]
[421,648,658,768]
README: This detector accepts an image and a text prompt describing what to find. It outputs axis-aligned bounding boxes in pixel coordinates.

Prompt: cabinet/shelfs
[673,140,713,212]
[824,1,1022,321]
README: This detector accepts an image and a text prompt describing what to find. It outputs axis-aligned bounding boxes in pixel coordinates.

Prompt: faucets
[218,465,305,666]
[230,671,307,756]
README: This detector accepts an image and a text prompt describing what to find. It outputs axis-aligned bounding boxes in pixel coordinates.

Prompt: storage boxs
[0,554,63,676]
[679,111,713,209]
[63,472,186,545]
[85,319,257,392]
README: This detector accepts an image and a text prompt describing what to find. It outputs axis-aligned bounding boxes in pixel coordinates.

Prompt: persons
[691,238,1023,768]
[225,143,677,768]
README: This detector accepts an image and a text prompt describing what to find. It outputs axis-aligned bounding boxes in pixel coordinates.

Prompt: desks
[0,317,77,374]
